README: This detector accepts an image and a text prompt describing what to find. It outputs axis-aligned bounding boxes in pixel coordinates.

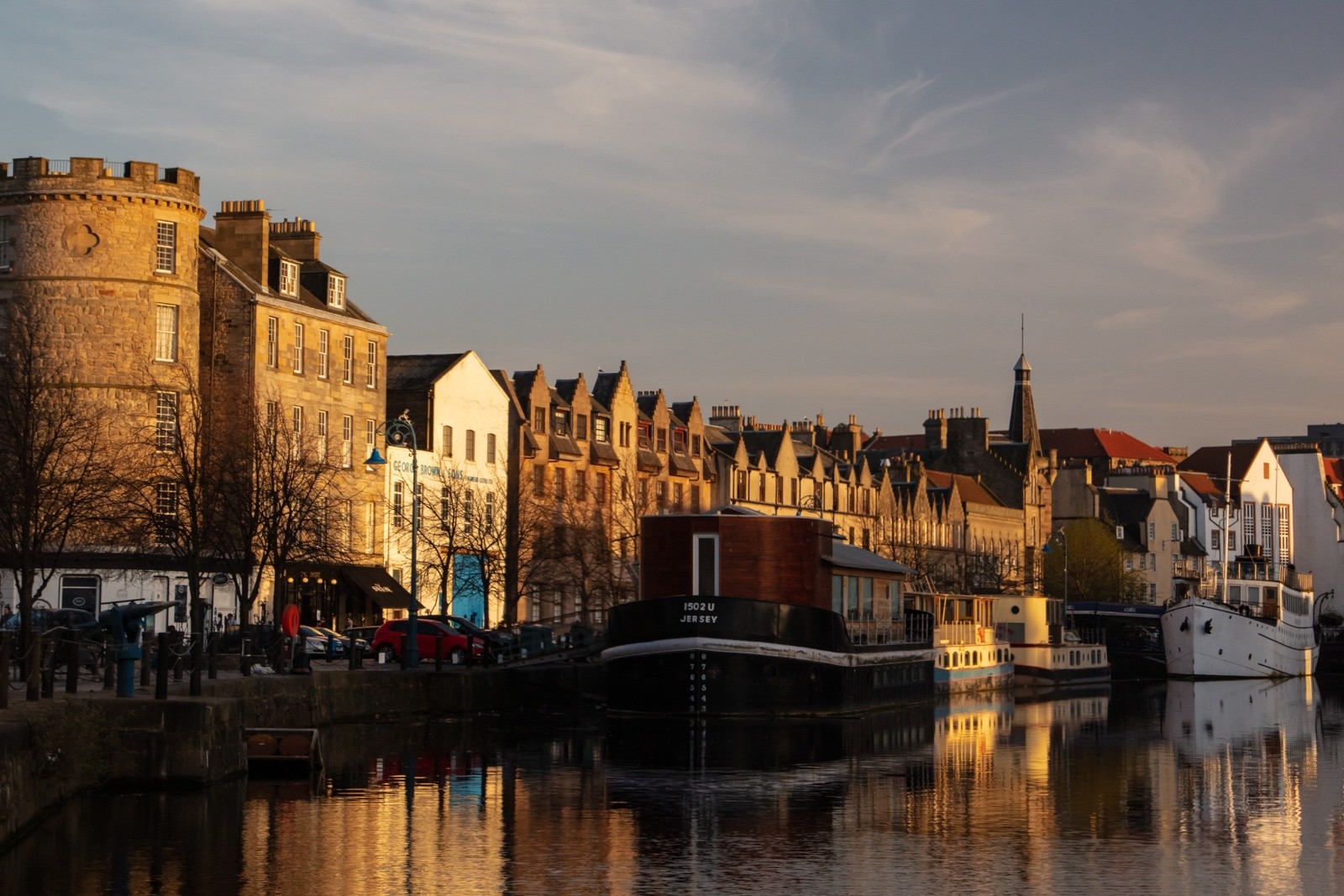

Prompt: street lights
[363,418,420,670]
[1040,531,1068,606]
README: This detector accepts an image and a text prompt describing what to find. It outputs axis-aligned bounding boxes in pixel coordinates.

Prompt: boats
[1161,453,1322,682]
[599,514,936,720]
[1066,602,1167,684]
[1163,675,1322,848]
[903,591,1015,696]
[974,593,1111,687]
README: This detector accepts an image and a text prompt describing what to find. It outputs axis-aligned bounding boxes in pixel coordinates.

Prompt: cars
[370,619,485,666]
[416,615,519,666]
[1,607,99,668]
[224,623,381,659]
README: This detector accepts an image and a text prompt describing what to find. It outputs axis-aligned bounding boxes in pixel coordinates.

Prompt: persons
[0,604,15,626]
[166,625,176,633]
[345,618,353,629]
[382,617,386,625]
[228,614,235,626]
[321,617,327,627]
